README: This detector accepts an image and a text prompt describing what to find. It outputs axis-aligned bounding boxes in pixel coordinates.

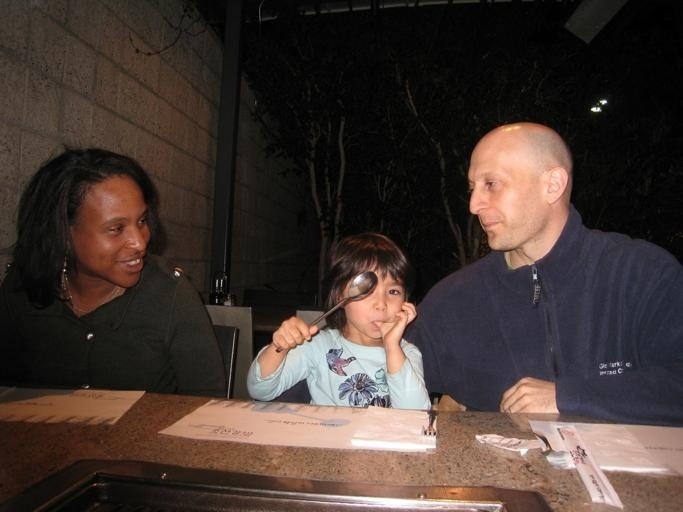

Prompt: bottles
[213,275,227,305]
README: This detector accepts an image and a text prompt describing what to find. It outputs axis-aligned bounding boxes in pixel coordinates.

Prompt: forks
[423,414,437,435]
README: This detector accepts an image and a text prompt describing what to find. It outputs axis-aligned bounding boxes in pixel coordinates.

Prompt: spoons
[275,270,378,353]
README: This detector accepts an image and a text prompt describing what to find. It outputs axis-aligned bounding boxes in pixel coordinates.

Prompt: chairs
[207,305,254,400]
[296,310,326,331]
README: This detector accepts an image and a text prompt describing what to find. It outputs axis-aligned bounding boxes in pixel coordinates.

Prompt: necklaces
[61,284,125,317]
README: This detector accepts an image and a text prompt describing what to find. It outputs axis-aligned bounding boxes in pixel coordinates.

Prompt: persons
[400,121,683,427]
[0,148,227,402]
[247,231,431,412]
